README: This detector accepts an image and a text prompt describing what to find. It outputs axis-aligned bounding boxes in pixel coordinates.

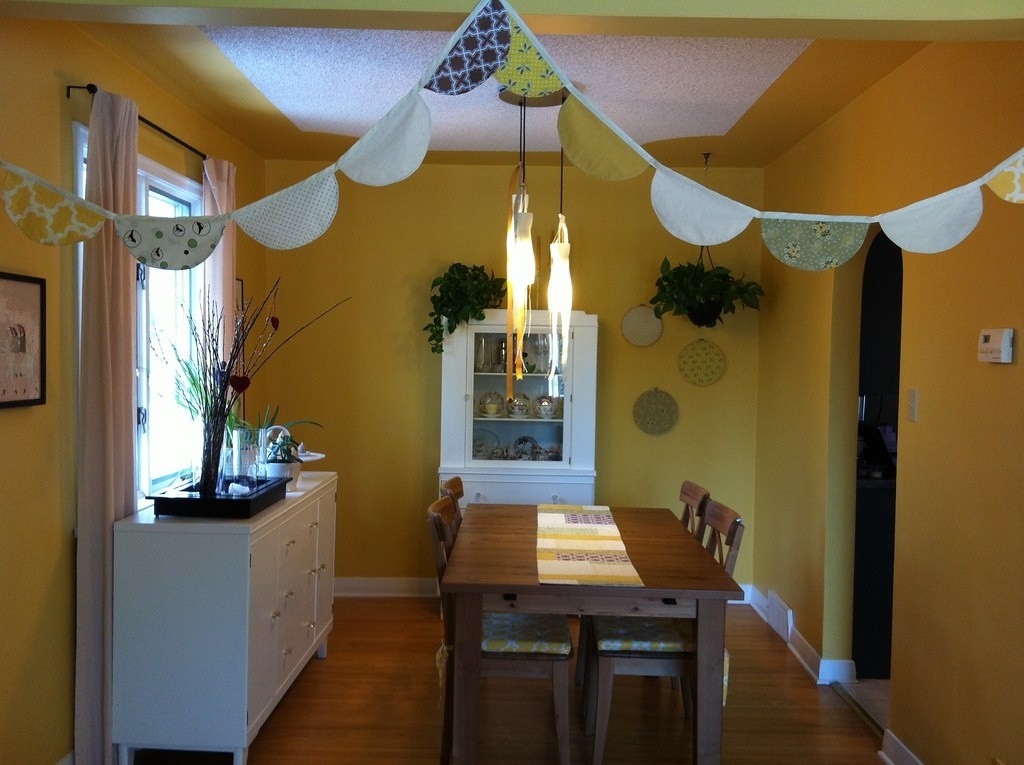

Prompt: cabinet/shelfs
[431,311,598,520]
[110,471,345,765]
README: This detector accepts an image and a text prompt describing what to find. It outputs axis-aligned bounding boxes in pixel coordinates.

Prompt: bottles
[496,340,506,371]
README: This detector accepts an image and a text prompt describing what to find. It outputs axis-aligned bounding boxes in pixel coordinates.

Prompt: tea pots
[502,443,521,460]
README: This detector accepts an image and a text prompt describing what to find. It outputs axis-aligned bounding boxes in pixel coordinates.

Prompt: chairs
[427,476,578,765]
[571,482,745,765]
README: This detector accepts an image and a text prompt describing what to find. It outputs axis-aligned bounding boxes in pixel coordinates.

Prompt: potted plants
[425,263,509,354]
[497,79,587,314]
[145,267,352,497]
[650,257,767,329]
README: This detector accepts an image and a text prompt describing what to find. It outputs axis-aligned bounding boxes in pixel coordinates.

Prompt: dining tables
[441,503,747,765]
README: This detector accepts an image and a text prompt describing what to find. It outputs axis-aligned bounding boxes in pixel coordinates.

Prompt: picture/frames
[1,271,47,410]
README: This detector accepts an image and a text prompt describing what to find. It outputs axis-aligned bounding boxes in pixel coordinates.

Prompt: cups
[491,450,502,459]
[509,404,527,414]
[233,427,267,487]
[536,405,555,414]
[478,404,498,413]
[520,453,532,461]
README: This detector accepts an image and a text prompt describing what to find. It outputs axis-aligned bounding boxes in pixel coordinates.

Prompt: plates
[507,393,530,413]
[536,414,557,419]
[513,436,538,453]
[480,392,506,412]
[533,394,556,413]
[479,414,504,418]
[508,413,531,418]
[473,429,500,459]
